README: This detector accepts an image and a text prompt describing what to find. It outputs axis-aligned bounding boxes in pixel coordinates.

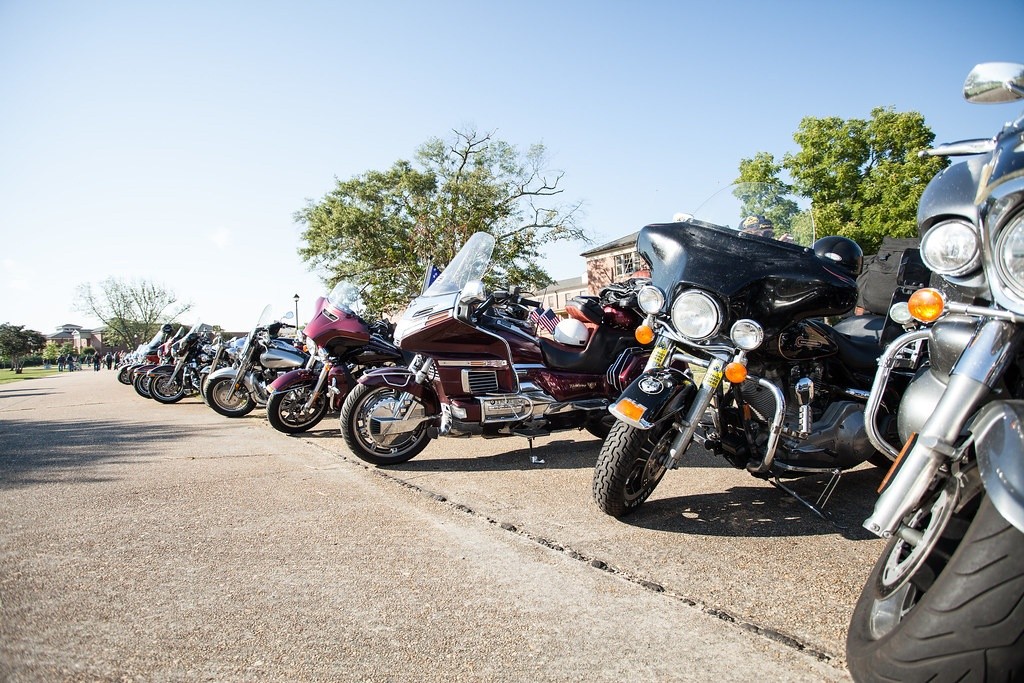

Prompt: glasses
[757,231,775,239]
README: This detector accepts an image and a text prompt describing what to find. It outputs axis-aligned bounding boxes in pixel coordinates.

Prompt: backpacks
[856,237,920,315]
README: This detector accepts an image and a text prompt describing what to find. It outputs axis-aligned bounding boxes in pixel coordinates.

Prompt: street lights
[293,294,300,330]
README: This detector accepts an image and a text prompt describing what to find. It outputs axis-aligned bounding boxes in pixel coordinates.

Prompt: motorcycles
[846,61,1024,683]
[266,280,413,435]
[594,215,916,519]
[117,303,323,419]
[340,231,694,467]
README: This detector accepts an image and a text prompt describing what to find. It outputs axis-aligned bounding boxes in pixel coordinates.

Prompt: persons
[56,350,126,371]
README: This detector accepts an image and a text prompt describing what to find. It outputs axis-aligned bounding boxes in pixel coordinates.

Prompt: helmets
[553,318,589,346]
[161,323,172,333]
[738,214,774,229]
[809,236,863,275]
